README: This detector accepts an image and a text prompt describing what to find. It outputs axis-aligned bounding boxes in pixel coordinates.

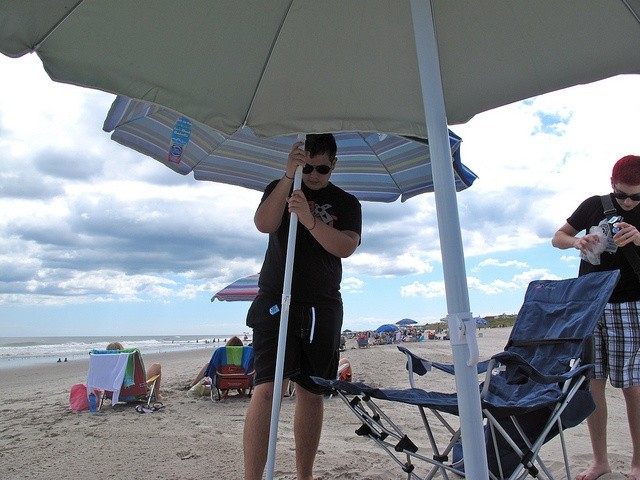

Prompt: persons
[189,336,255,399]
[552,154,640,480]
[243,132,364,480]
[336,357,352,380]
[106,342,161,402]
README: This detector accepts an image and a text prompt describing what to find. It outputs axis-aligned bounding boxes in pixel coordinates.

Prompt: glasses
[303,163,334,174]
[613,187,640,201]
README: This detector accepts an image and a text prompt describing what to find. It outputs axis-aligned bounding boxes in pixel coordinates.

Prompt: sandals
[135,403,153,413]
[151,401,166,412]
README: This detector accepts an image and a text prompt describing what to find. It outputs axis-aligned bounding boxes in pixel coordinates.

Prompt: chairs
[90,349,160,410]
[310,265,622,479]
[290,363,349,401]
[213,346,256,400]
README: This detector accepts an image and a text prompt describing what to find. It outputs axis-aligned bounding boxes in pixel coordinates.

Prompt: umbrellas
[374,323,399,334]
[0,0,640,480]
[439,314,449,323]
[343,328,352,337]
[209,269,272,305]
[473,315,487,338]
[102,89,480,480]
[396,318,417,330]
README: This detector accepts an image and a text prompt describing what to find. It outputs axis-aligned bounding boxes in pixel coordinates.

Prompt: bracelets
[280,169,296,183]
[306,214,320,233]
